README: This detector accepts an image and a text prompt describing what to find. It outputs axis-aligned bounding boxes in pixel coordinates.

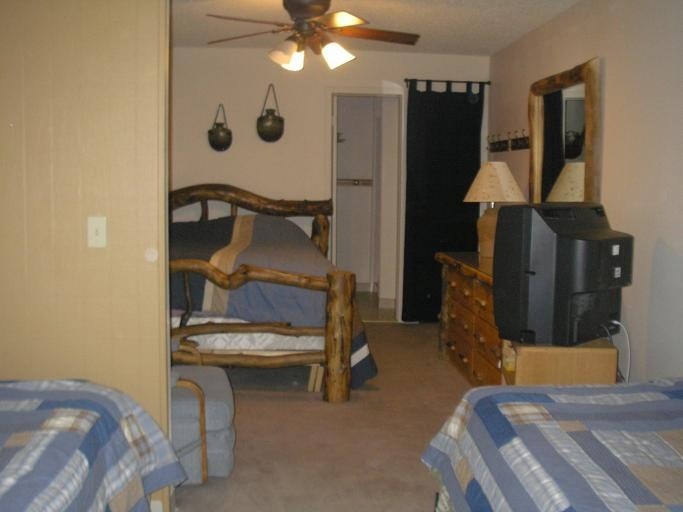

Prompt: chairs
[167,347,237,484]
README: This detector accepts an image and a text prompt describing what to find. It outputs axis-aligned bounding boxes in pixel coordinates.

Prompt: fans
[206,1,420,47]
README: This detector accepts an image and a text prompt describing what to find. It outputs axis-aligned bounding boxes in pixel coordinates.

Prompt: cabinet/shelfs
[432,250,499,389]
[500,338,618,386]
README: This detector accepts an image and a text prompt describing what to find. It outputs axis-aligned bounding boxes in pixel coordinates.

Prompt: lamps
[463,160,526,255]
[546,160,585,201]
[269,12,369,71]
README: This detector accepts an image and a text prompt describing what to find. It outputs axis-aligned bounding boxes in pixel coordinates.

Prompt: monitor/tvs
[492,201,634,347]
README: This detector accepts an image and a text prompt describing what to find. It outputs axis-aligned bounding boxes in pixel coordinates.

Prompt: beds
[169,183,378,403]
[421,371,682,512]
[0,377,188,512]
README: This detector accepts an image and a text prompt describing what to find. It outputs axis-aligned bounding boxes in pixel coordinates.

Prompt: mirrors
[523,53,602,207]
